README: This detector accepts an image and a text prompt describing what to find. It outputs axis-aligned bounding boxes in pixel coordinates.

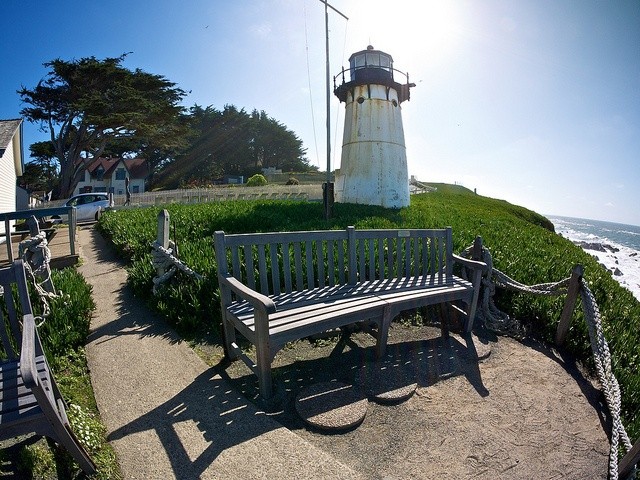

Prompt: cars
[46,193,114,227]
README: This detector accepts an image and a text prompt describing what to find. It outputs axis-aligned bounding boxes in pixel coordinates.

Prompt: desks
[13,219,56,240]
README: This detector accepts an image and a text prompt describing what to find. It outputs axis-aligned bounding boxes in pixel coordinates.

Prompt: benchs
[0,228,58,246]
[0,258,104,475]
[214,225,490,398]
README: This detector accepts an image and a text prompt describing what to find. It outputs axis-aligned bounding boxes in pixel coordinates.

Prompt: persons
[123,177,132,206]
